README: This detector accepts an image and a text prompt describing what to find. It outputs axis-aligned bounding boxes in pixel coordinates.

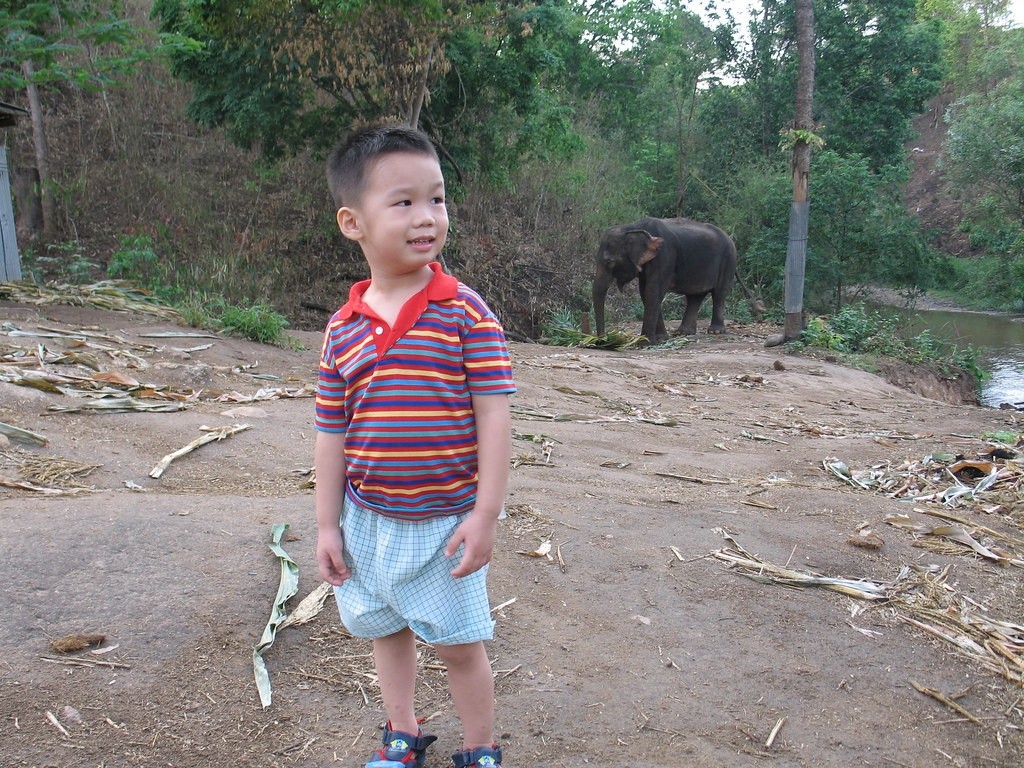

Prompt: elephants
[592,216,738,347]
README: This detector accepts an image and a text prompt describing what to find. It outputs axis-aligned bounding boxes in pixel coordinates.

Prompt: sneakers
[451,744,504,768]
[365,717,438,768]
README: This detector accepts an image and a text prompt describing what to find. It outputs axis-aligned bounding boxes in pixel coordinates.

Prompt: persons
[312,122,518,768]
